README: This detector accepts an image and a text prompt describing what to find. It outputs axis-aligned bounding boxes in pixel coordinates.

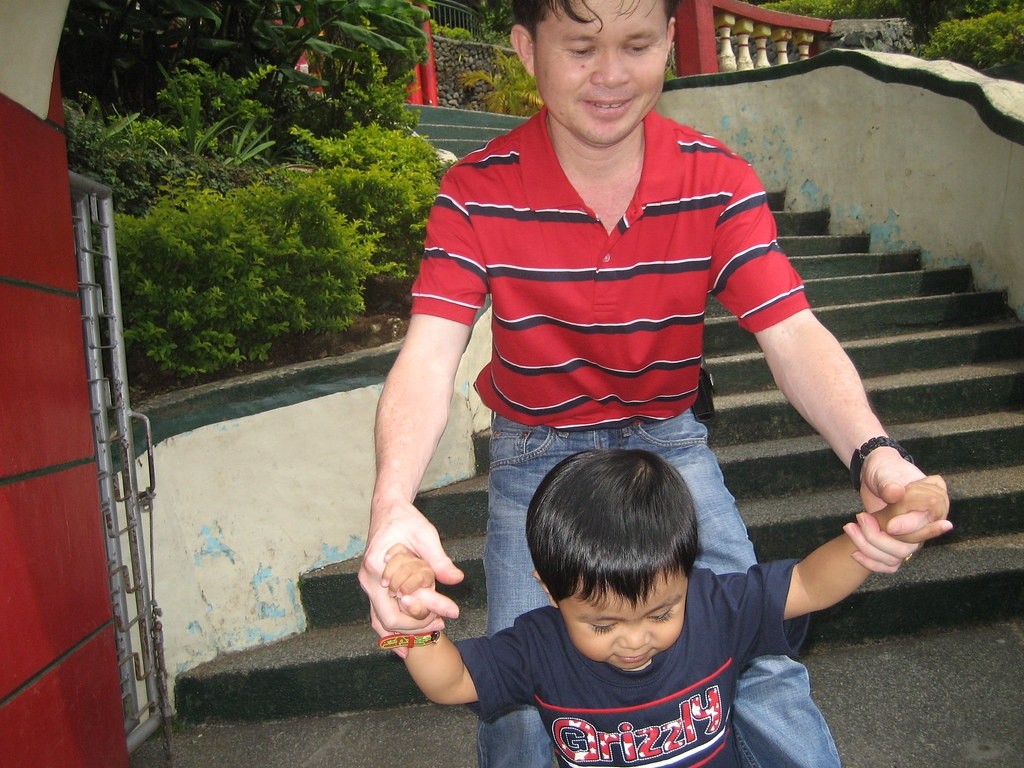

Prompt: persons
[382,448,954,766]
[357,0,954,767]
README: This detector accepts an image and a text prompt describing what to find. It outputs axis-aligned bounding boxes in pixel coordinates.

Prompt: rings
[903,552,914,562]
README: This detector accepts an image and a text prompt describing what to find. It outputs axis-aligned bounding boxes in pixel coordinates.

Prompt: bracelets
[377,627,440,651]
[850,435,914,492]
